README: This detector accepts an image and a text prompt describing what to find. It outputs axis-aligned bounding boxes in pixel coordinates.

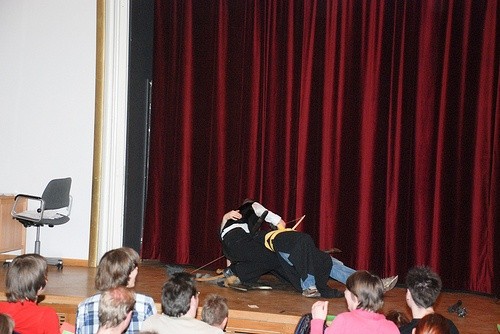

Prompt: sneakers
[381,274,398,296]
[301,287,320,297]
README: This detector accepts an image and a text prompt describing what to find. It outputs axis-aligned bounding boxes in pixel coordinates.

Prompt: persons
[311,270,400,334]
[0,314,14,334]
[218,199,399,297]
[400,265,459,334]
[141,271,224,334]
[76,247,158,334]
[0,253,61,334]
[411,313,451,334]
[95,287,137,334]
[200,294,229,329]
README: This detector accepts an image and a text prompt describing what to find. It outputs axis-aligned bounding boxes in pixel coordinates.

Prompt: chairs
[3,177,73,271]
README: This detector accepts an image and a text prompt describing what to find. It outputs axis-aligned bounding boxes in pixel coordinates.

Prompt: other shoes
[243,279,273,290]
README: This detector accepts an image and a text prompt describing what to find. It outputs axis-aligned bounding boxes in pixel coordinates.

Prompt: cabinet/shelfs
[0,194,26,266]
